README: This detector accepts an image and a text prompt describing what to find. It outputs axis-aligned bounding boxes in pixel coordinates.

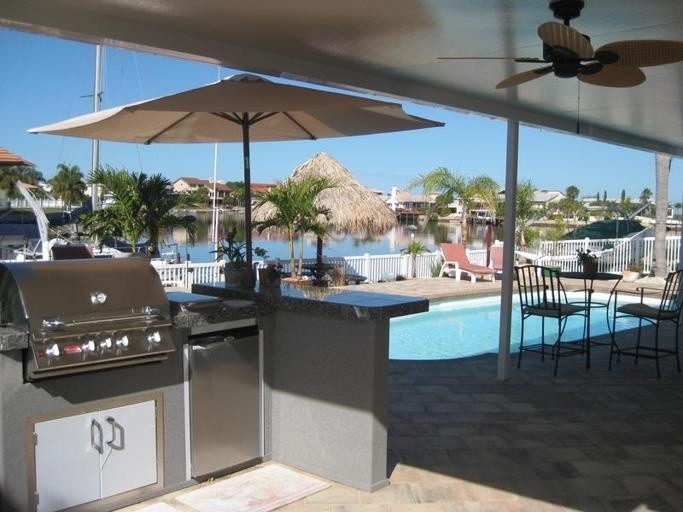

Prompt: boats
[467,209,504,225]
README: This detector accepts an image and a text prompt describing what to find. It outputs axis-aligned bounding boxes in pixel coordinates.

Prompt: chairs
[427,240,529,284]
[515,250,680,382]
[9,238,175,270]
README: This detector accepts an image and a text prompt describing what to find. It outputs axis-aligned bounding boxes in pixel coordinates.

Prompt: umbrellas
[26,73,447,288]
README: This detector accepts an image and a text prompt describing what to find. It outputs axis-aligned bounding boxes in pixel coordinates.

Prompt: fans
[435,0,680,100]
